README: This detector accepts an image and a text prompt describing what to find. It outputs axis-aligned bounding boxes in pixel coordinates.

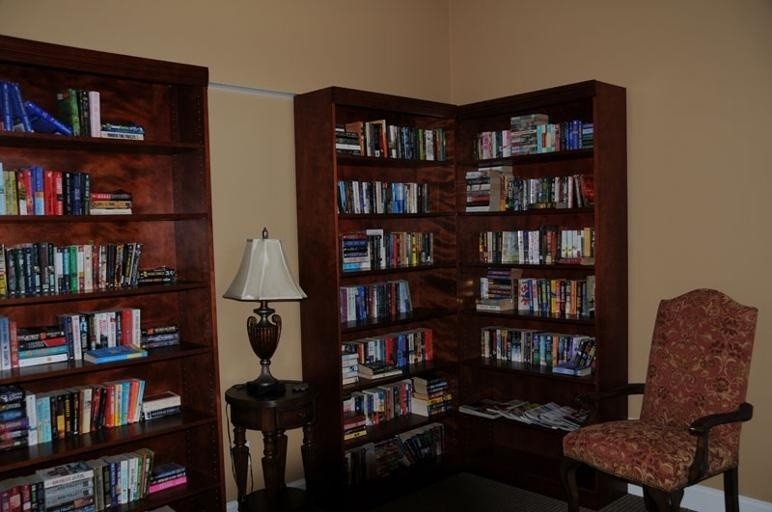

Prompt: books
[475,266,596,322]
[480,326,596,377]
[0,81,145,141]
[479,227,596,266]
[340,229,434,272]
[337,180,428,214]
[465,166,595,212]
[2,308,180,371]
[1,448,187,512]
[460,398,586,432]
[345,423,446,481]
[1,376,181,447]
[1,162,133,215]
[335,120,445,162]
[341,327,433,384]
[344,375,453,439]
[1,239,175,297]
[477,113,596,160]
[340,280,415,321]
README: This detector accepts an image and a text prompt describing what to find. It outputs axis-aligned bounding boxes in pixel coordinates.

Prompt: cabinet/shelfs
[294,85,458,512]
[459,79,627,511]
[0,33,226,511]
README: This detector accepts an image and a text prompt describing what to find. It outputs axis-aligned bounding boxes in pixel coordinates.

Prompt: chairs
[562,289,758,512]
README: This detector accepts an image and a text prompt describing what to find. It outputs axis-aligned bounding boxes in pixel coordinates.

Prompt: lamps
[222,227,309,395]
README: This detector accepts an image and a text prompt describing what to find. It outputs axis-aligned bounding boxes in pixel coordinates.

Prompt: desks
[226,380,319,512]
